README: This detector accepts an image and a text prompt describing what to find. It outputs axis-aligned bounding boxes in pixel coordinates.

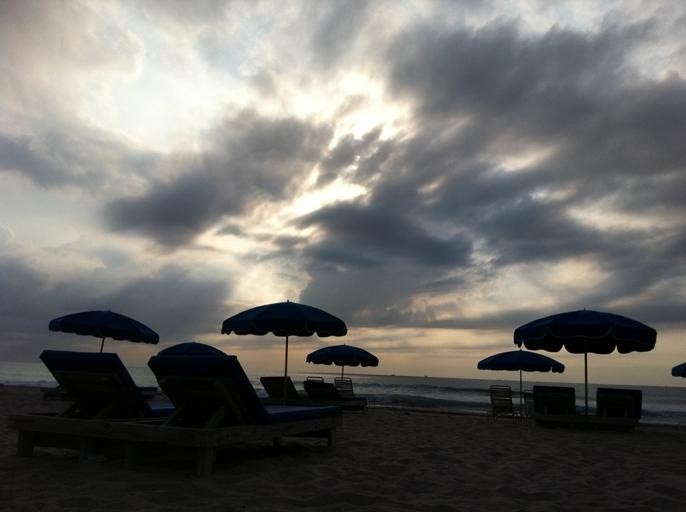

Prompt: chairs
[488,384,643,433]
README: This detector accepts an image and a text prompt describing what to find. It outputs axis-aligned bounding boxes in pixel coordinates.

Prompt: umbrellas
[157,340,229,357]
[514,306,658,417]
[304,343,378,391]
[670,362,686,378]
[477,350,565,413]
[221,298,348,404]
[48,309,160,352]
